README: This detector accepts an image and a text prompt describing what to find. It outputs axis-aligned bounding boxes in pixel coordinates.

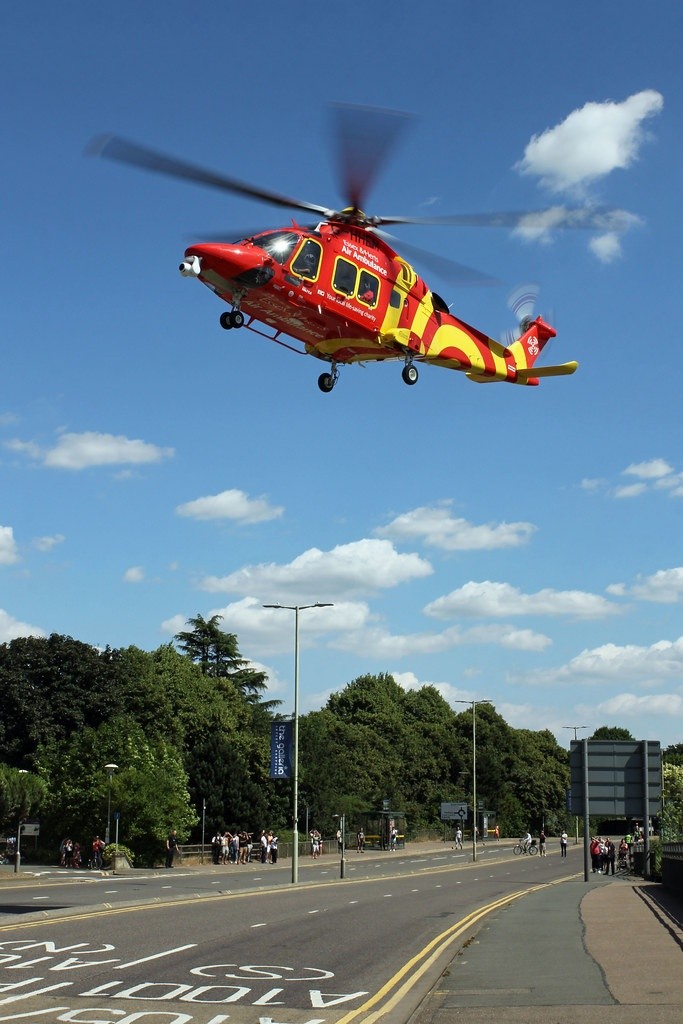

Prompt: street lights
[562,726,589,844]
[104,764,118,846]
[15,770,28,873]
[455,700,492,862]
[263,601,334,882]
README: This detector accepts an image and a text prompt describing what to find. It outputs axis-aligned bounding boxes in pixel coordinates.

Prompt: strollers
[616,850,628,871]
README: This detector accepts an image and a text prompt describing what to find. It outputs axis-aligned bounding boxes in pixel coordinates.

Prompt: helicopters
[82,105,624,392]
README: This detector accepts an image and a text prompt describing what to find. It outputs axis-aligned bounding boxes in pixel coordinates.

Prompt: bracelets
[296,269,299,273]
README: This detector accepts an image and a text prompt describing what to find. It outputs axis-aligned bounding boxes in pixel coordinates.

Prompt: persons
[357,827,365,853]
[618,831,644,876]
[495,826,499,840]
[165,830,181,867]
[293,254,315,279]
[309,829,323,860]
[337,828,347,853]
[539,830,546,857]
[560,830,568,858]
[260,829,278,864]
[60,837,82,868]
[521,830,532,853]
[389,827,397,852]
[358,279,374,305]
[455,826,462,849]
[93,836,103,868]
[635,823,639,831]
[211,831,254,864]
[591,836,615,875]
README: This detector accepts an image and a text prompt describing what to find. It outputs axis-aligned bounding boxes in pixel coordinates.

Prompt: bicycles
[513,839,538,856]
[91,851,102,869]
